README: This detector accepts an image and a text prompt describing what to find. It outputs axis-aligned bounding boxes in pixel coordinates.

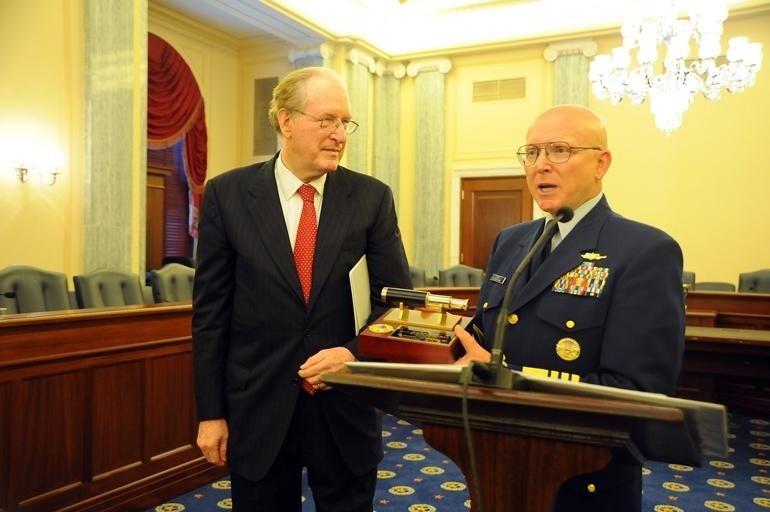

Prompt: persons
[476,105,684,511]
[191,67,414,511]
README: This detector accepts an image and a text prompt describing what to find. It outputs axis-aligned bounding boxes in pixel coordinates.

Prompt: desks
[315,359,704,512]
[0,300,231,511]
[413,281,481,320]
[677,291,770,411]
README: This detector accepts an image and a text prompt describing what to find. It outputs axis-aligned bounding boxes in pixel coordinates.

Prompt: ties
[528,220,559,279]
[293,184,317,394]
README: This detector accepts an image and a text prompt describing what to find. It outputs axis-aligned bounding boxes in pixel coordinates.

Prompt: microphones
[456,206,573,389]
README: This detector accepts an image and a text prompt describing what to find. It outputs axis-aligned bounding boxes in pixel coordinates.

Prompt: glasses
[515,142,600,167]
[289,108,358,134]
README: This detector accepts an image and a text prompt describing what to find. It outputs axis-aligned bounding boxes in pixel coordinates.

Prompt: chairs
[407,266,427,290]
[72,269,149,312]
[694,282,740,293]
[682,270,696,290]
[0,262,71,318]
[152,263,198,307]
[740,268,770,293]
[437,265,486,290]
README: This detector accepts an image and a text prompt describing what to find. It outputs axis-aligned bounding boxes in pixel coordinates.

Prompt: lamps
[586,0,768,132]
[0,116,67,186]
